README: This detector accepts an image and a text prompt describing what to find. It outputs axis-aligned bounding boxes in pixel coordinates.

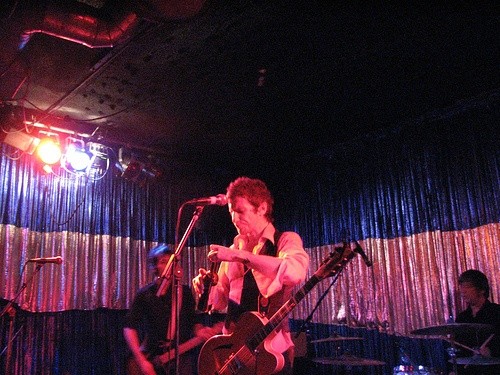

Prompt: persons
[192,177,309,375]
[123,245,196,375]
[441,269,500,358]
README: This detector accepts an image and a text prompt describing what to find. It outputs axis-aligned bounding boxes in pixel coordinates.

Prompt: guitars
[126,321,224,375]
[198,246,356,375]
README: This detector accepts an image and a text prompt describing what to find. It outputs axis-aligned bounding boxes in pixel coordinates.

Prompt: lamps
[0,106,161,188]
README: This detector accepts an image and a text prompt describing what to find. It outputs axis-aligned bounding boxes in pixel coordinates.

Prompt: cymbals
[410,322,497,336]
[309,336,367,344]
[447,357,499,366]
[312,354,387,366]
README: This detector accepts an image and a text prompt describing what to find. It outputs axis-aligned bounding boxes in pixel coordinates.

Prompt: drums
[390,365,445,375]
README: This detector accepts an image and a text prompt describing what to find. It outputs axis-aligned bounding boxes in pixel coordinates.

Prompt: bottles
[393,365,433,375]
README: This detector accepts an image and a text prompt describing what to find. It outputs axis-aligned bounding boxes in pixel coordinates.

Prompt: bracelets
[243,258,249,263]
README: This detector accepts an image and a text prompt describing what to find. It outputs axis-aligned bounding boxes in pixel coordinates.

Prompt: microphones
[28,256,63,264]
[353,238,372,267]
[185,194,228,206]
